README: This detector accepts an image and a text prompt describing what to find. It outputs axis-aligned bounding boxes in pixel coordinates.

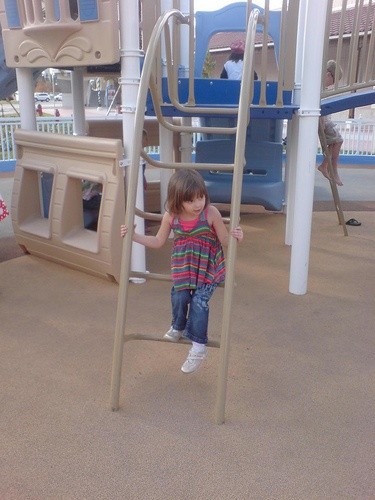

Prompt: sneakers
[181,348,208,374]
[165,326,185,342]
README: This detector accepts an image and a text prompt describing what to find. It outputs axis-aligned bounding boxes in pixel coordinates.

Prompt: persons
[220,40,258,80]
[318,60,344,186]
[120,169,244,374]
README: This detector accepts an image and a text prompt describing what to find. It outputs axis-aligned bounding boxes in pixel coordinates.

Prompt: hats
[230,40,245,54]
[326,60,344,84]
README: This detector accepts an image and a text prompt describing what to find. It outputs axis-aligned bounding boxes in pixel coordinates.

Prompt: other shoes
[345,218,361,226]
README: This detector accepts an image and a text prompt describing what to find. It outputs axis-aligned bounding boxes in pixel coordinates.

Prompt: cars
[33,93,62,102]
